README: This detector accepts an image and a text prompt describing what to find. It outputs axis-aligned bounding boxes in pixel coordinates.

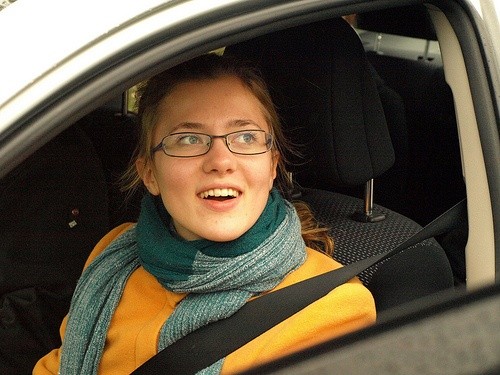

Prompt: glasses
[153,130,277,158]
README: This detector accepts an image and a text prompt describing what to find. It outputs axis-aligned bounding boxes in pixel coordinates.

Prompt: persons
[32,50,376,375]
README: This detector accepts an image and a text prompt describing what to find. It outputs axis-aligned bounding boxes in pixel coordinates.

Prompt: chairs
[295,3,468,290]
[222,15,458,318]
[78,86,149,230]
